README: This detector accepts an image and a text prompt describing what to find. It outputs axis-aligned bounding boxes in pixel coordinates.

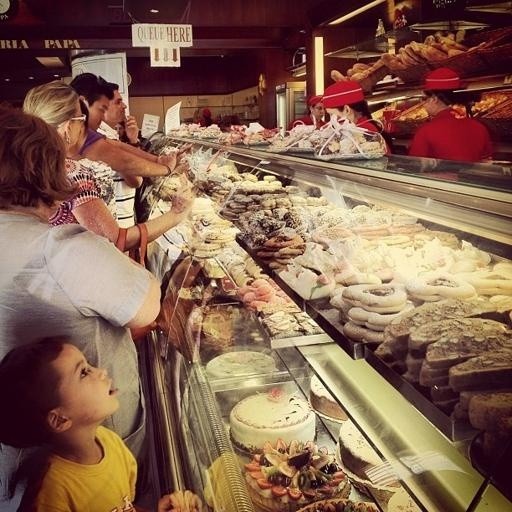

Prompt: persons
[407,67,494,162]
[193,107,214,126]
[287,95,328,133]
[321,79,395,156]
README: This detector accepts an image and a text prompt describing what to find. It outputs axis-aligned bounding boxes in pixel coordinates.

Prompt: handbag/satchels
[114,223,158,344]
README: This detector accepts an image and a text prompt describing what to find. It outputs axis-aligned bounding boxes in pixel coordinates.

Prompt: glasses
[71,114,87,122]
[113,126,123,130]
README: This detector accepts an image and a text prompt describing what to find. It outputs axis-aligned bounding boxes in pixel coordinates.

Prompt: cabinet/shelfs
[134,133,512,512]
[180,105,260,123]
[324,0,512,101]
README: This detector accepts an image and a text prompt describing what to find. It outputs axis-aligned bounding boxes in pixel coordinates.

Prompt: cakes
[207,351,423,512]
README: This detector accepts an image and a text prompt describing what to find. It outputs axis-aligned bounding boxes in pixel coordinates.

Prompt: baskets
[385,26,512,85]
[390,88,512,140]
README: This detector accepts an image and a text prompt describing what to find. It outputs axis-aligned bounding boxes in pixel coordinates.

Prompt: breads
[329,32,484,84]
[156,119,512,461]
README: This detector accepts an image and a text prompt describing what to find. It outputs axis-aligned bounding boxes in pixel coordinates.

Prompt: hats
[421,66,463,96]
[307,94,322,107]
[202,109,212,118]
[323,80,366,110]
